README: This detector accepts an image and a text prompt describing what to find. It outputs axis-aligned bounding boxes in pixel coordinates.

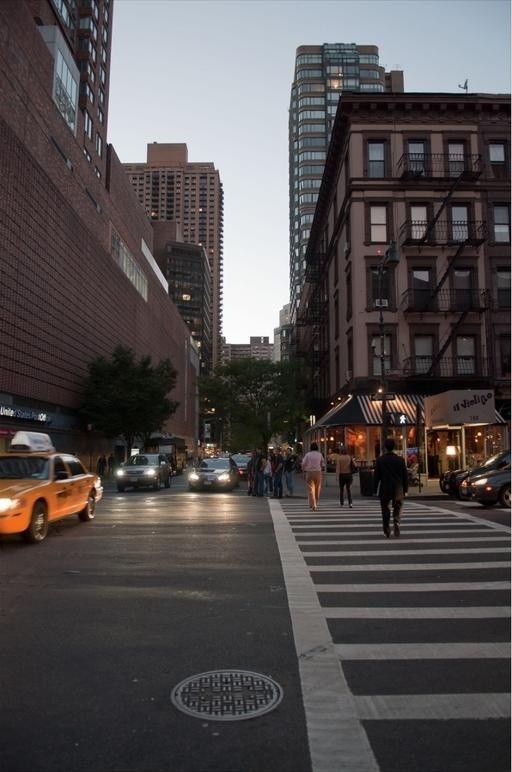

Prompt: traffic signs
[371,392,396,401]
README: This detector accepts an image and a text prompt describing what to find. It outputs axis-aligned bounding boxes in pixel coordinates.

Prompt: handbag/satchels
[350,462,357,473]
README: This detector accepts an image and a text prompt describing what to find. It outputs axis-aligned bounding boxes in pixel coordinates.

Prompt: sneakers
[394,523,400,537]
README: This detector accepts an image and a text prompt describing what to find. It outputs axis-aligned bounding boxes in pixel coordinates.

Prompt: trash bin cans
[358,469,380,498]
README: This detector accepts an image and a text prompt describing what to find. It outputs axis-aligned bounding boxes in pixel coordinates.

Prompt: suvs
[115,453,174,493]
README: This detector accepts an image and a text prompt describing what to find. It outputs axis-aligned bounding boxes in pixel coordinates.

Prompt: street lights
[378,240,399,459]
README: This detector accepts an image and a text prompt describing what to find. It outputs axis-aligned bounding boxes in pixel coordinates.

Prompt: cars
[459,460,510,509]
[0,432,104,543]
[440,449,511,500]
[188,457,240,492]
[231,453,256,479]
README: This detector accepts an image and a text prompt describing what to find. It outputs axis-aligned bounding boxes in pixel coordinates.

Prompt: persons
[96,442,340,511]
[372,439,409,538]
[336,447,355,508]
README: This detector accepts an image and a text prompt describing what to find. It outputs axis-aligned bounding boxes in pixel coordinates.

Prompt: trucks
[142,438,189,476]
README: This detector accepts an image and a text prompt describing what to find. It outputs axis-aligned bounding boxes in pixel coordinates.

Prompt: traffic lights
[395,412,406,425]
